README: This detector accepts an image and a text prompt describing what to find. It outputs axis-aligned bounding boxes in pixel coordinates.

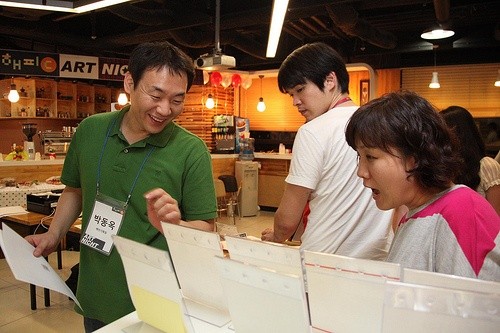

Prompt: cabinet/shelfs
[0,75,112,120]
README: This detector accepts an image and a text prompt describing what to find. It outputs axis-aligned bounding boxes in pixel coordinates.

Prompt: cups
[35,152,41,160]
[63,127,70,137]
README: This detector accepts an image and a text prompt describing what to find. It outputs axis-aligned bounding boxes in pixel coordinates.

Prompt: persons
[435,106,500,217]
[23,38,220,333]
[260,42,409,262]
[345,91,500,283]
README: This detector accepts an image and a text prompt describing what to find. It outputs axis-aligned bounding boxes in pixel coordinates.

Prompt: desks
[0,182,66,208]
[1,211,83,311]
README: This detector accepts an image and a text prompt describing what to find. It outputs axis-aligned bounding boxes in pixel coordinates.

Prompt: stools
[214,175,240,226]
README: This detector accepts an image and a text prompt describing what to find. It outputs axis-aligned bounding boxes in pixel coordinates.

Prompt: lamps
[421,24,456,40]
[429,43,440,88]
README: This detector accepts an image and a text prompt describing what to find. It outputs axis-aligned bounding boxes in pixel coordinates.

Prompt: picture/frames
[360,80,369,107]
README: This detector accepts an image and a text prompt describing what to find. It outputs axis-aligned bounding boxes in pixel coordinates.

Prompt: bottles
[279,144,285,154]
[17,85,107,118]
[239,137,255,161]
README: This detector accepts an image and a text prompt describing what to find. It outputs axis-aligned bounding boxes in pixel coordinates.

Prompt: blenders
[22,123,38,160]
